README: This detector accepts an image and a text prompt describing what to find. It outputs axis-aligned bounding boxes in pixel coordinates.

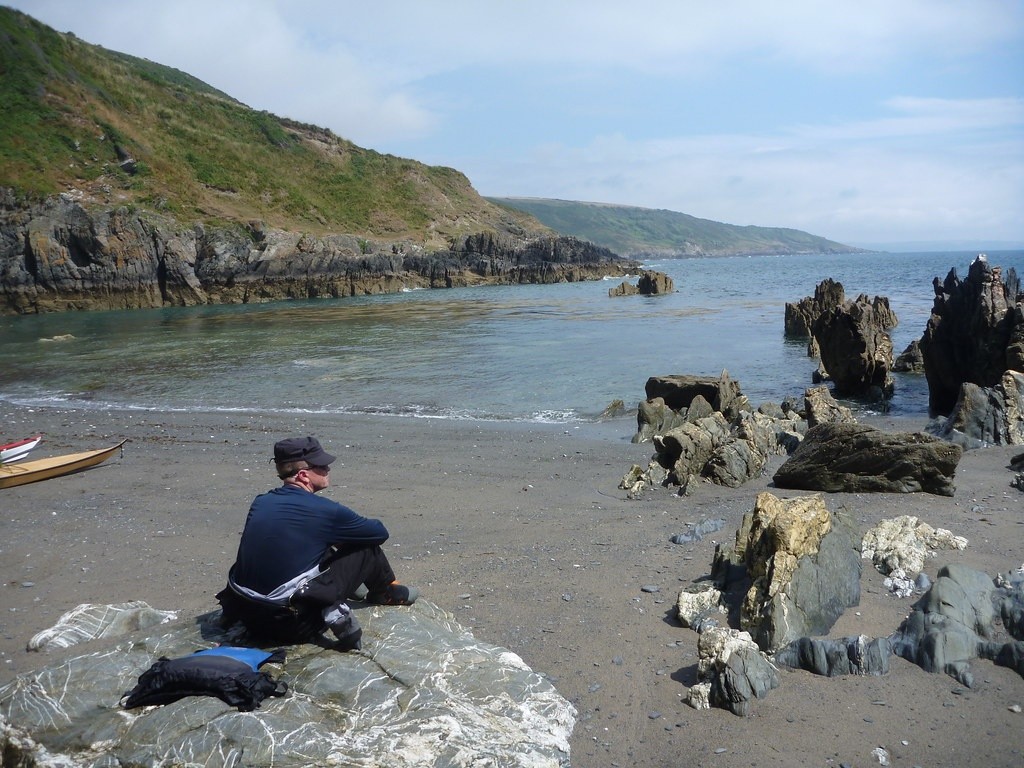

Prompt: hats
[268,437,337,466]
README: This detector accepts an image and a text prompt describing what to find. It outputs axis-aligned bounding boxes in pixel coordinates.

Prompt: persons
[217,437,418,643]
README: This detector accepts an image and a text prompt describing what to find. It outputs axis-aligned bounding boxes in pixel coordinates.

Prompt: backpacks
[118,656,288,713]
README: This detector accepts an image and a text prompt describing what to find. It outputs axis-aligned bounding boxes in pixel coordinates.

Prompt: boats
[0,433,44,465]
[0,438,129,489]
